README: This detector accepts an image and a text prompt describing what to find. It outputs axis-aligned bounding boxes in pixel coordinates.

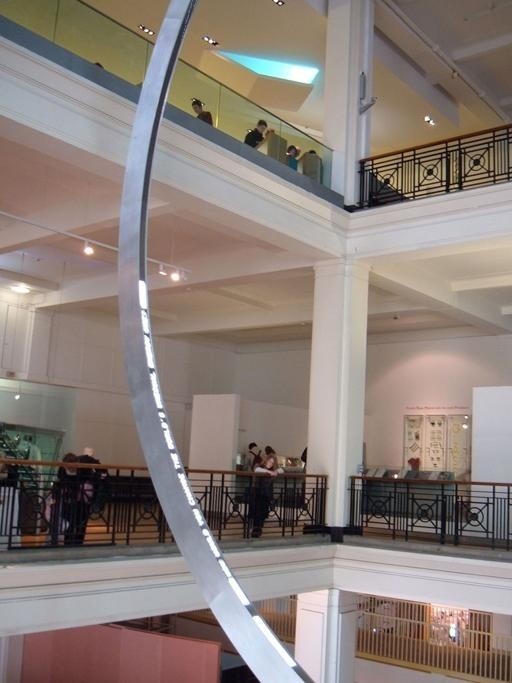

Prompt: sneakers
[251,528,263,537]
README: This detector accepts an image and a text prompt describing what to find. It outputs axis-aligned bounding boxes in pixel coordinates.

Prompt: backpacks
[248,450,264,472]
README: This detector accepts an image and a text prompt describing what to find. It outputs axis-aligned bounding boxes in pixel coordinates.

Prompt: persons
[245,443,266,492]
[301,447,307,471]
[57,453,112,544]
[286,145,316,158]
[245,119,267,148]
[44,481,72,546]
[16,435,42,471]
[250,454,285,537]
[192,100,212,125]
[265,445,278,516]
